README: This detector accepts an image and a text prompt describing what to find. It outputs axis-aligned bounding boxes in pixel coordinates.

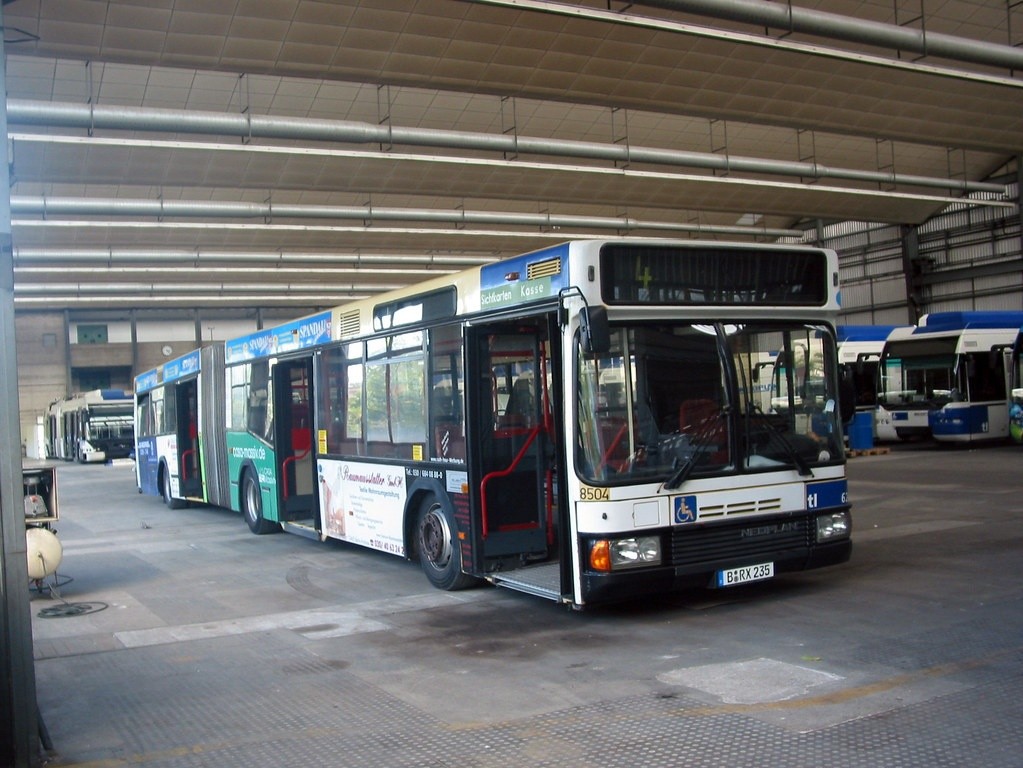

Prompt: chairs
[679,398,727,464]
[291,404,308,428]
[435,424,464,458]
[599,416,639,470]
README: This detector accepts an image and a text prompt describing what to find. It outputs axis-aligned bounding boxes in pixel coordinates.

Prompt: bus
[988,326,1023,446]
[42,389,132,465]
[750,324,913,458]
[132,237,854,612]
[856,310,1023,449]
[431,331,827,420]
